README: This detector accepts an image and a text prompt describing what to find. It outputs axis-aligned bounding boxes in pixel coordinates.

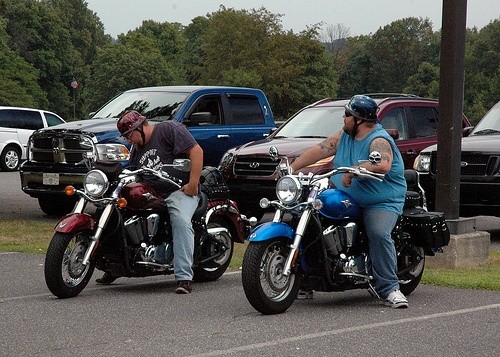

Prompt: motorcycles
[44,152,258,299]
[241,145,451,315]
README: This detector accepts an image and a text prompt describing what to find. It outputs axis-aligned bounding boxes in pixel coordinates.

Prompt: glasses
[345,111,354,117]
[123,131,134,141]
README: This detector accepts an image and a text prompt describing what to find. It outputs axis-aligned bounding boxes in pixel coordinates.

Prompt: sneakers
[95,272,118,284]
[296,290,314,299]
[382,289,409,309]
[174,280,191,294]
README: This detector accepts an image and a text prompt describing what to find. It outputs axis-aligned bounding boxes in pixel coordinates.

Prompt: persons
[96,111,203,293]
[276,94,408,308]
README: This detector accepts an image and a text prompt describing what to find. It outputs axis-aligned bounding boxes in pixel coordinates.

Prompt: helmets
[117,111,147,137]
[344,95,378,121]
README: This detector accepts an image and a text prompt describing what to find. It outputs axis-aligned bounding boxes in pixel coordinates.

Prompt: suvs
[18,85,279,215]
[218,93,475,223]
[412,99,500,217]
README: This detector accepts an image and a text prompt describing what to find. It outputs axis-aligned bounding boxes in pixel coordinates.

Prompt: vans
[0,106,67,172]
